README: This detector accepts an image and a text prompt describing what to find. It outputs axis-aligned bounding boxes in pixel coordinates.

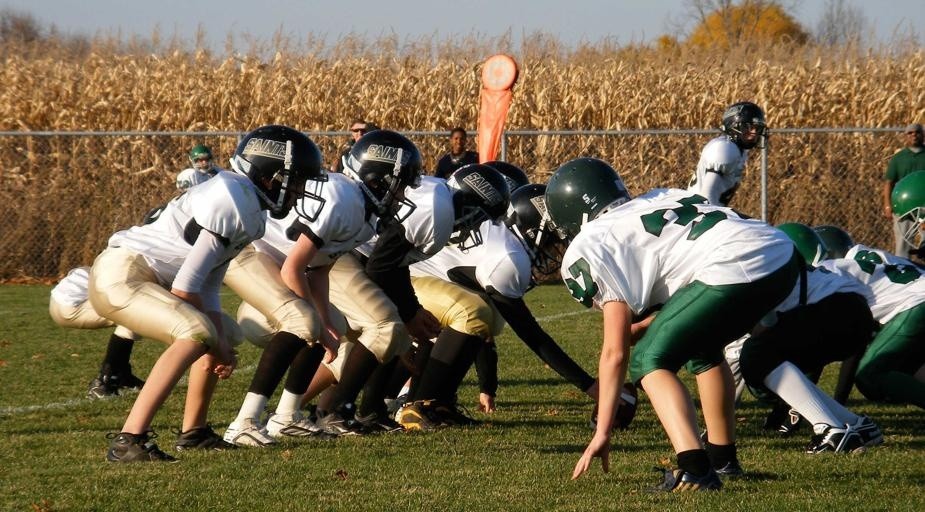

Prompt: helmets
[446,157,628,291]
[189,146,214,175]
[719,102,770,150]
[776,223,855,270]
[341,129,424,223]
[891,170,925,250]
[229,124,329,222]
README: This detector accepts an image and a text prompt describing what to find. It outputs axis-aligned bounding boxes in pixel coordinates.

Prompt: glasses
[351,129,366,132]
[907,130,920,134]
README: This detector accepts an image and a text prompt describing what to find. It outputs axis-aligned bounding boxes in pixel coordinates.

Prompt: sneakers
[265,395,479,443]
[171,425,238,456]
[222,420,278,448]
[643,459,743,495]
[86,374,145,404]
[105,431,181,464]
[803,411,885,456]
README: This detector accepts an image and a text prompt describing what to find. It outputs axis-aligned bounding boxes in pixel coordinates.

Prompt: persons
[48,103,925,492]
